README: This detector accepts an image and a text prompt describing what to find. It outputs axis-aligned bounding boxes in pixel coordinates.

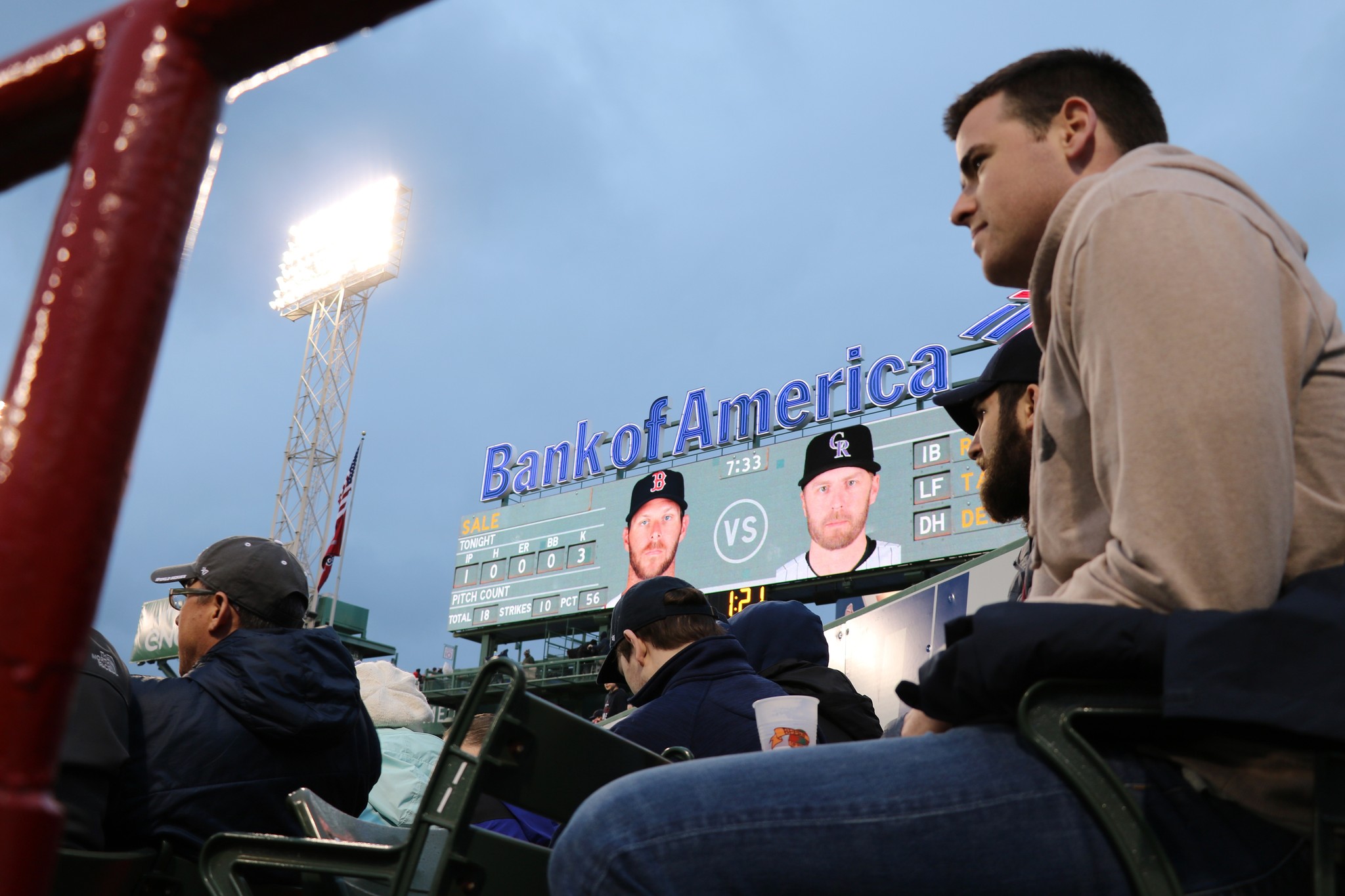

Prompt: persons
[476,326,1051,845]
[413,632,536,681]
[775,424,902,583]
[349,659,444,827]
[549,48,1345,896]
[600,469,689,608]
[129,537,382,893]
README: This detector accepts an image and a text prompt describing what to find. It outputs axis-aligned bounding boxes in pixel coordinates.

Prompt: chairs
[55,659,1345,896]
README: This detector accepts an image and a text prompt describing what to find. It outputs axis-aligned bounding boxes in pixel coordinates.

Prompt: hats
[151,535,309,629]
[355,660,436,729]
[596,576,728,684]
[626,469,688,522]
[798,425,881,490]
[932,327,1043,436]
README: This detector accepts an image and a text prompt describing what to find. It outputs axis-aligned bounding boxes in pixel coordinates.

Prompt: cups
[752,695,820,751]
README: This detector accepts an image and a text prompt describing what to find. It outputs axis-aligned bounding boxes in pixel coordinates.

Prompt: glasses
[169,588,215,611]
